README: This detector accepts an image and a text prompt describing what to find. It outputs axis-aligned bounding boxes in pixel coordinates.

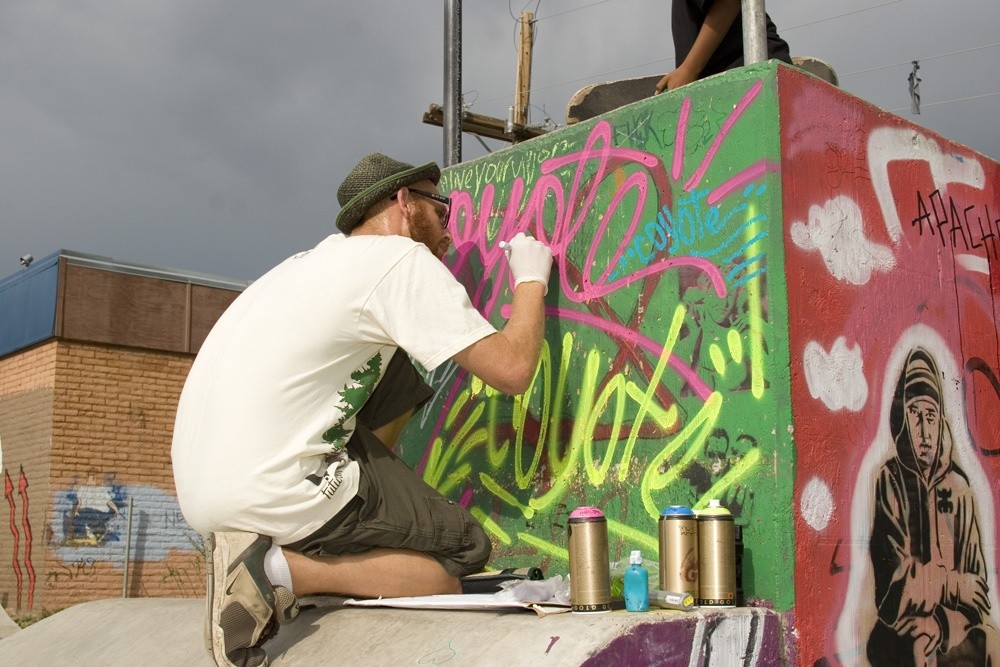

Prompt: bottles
[624,551,649,612]
[659,506,699,605]
[735,526,744,606]
[698,500,738,608]
[569,507,612,614]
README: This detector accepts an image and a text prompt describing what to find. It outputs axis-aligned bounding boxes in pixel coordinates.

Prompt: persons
[653,0,793,95]
[170,146,553,667]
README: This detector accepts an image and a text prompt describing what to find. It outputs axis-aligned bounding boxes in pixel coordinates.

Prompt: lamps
[19,254,34,266]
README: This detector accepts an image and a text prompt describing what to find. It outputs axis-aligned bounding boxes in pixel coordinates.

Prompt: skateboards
[565,55,840,126]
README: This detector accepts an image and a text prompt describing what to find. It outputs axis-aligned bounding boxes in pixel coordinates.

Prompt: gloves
[504,232,554,296]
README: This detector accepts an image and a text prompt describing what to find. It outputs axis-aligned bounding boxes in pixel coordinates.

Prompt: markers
[498,241,512,251]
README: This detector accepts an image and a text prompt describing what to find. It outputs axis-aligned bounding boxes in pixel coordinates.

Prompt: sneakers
[205,530,301,667]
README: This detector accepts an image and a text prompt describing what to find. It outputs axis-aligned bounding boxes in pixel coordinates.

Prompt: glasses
[389,187,453,229]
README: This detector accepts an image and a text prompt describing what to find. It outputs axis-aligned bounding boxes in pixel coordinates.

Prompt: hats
[334,152,441,234]
